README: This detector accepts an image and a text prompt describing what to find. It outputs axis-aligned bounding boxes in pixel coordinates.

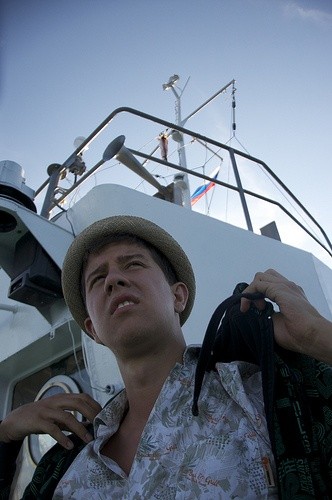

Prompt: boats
[0,73,332,500]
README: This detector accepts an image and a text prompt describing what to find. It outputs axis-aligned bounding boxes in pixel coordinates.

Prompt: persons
[0,215,332,500]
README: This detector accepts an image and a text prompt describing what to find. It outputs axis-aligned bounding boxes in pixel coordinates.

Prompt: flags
[191,165,220,206]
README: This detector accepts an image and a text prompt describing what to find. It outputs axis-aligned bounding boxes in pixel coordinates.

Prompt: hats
[62,215,196,347]
